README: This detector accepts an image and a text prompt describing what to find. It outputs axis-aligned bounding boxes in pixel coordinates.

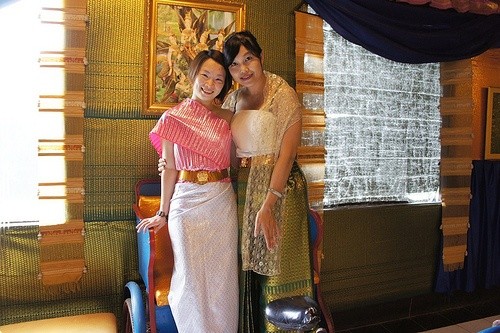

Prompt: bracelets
[268,188,286,199]
[155,210,169,222]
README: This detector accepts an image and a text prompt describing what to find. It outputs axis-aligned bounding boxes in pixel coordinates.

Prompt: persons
[135,50,246,333]
[157,32,316,333]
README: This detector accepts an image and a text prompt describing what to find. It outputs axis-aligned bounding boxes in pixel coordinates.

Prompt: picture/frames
[142,0,247,116]
[485,87,500,160]
